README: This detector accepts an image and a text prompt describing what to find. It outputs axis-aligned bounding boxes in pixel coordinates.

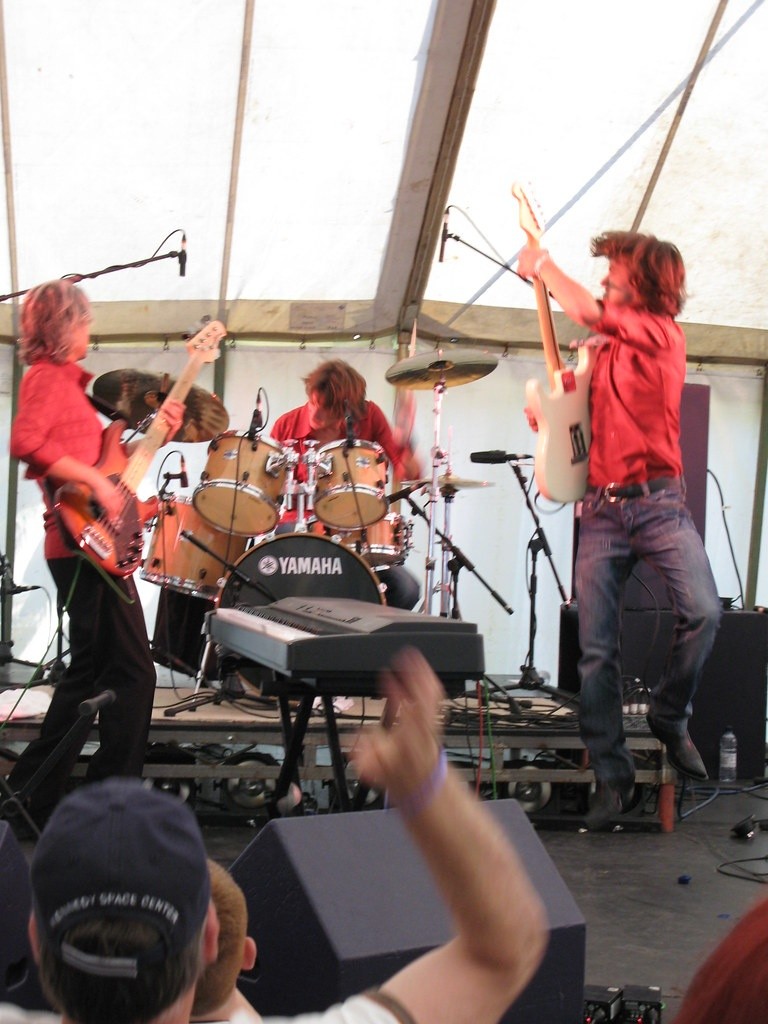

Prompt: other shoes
[646,710,708,781]
[574,778,643,832]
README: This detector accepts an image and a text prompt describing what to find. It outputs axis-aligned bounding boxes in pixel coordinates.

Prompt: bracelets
[533,254,551,278]
[396,744,448,821]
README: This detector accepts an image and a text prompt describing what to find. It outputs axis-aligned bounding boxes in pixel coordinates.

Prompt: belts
[585,476,670,502]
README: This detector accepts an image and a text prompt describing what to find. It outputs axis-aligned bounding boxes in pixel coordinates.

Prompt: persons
[269,359,421,611]
[0,644,551,1024]
[516,230,722,833]
[0,279,188,844]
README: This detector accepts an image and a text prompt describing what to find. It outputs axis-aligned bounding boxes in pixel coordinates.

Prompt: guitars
[54,319,228,574]
[512,178,595,505]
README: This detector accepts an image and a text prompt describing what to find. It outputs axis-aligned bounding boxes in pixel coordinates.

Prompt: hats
[30,779,212,981]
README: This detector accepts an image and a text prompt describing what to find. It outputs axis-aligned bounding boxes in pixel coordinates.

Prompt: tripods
[465,461,580,706]
[164,530,315,718]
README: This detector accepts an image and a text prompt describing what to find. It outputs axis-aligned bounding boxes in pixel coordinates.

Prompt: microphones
[386,482,428,503]
[180,234,186,277]
[181,455,188,487]
[10,586,40,594]
[439,209,449,262]
[470,450,533,464]
[255,391,262,427]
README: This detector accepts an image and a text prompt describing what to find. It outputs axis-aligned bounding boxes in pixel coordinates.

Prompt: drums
[192,430,289,538]
[141,495,245,601]
[213,532,387,697]
[307,510,407,567]
[313,438,389,532]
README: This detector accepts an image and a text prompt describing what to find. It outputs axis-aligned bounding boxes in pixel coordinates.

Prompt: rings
[177,420,182,424]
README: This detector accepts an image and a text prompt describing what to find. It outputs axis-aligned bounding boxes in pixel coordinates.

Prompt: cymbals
[92,368,229,444]
[384,348,498,391]
[398,475,496,489]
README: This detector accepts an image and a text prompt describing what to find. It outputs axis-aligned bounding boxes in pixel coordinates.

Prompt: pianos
[202,593,488,812]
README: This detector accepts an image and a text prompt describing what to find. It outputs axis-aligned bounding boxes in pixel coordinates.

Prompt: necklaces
[313,438,319,450]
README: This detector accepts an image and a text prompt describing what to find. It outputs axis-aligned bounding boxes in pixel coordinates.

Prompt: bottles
[719,725,737,781]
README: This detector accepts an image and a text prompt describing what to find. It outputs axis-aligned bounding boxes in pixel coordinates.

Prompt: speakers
[224,798,586,1024]
[0,821,63,1015]
[557,601,768,779]
